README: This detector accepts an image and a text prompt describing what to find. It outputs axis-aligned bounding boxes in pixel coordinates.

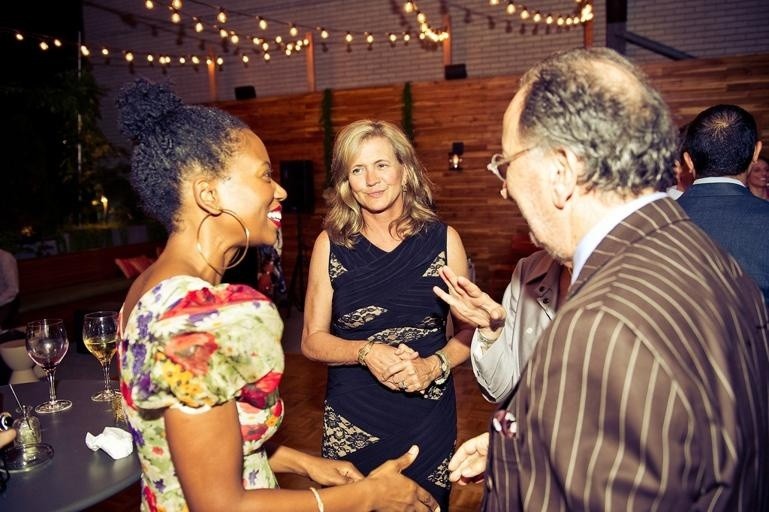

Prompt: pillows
[111,249,152,279]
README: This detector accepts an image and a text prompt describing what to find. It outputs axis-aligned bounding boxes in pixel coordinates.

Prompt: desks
[0,373,152,511]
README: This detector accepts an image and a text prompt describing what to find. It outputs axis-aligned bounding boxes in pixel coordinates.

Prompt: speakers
[280,158,316,214]
[445,63,467,80]
[235,85,256,99]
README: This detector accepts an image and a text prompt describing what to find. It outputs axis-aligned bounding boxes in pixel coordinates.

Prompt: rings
[399,381,408,389]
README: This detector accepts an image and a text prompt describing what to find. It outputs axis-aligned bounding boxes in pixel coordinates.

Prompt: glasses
[486,145,540,181]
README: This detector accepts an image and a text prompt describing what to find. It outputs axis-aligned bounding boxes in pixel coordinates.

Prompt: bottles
[11,404,42,449]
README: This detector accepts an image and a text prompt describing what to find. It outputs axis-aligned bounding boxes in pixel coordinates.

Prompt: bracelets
[433,350,450,385]
[309,486,324,512]
[477,327,503,344]
[357,340,375,367]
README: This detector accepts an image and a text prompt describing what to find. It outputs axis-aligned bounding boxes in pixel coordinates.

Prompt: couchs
[4,244,184,330]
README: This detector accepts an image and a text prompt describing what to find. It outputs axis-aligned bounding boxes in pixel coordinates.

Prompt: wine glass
[83,311,122,403]
[26,317,73,415]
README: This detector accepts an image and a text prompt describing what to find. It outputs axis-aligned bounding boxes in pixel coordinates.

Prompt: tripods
[286,214,310,318]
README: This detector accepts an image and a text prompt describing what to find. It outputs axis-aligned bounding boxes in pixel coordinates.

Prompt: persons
[112,76,441,512]
[662,103,768,305]
[432,246,576,406]
[447,48,767,512]
[0,248,21,331]
[300,119,477,512]
